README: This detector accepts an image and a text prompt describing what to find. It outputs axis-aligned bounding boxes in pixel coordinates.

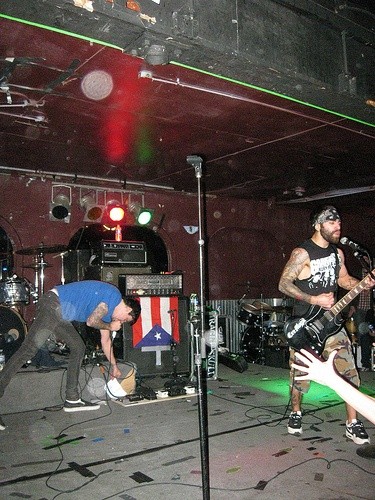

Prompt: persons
[278,206,375,445]
[347,254,375,374]
[0,280,141,431]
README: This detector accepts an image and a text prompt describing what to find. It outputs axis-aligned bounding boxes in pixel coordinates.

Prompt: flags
[132,296,179,350]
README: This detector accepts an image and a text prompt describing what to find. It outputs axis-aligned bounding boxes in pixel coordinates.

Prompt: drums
[253,301,273,326]
[0,277,30,307]
[241,327,262,364]
[238,303,256,326]
[264,319,284,336]
[0,305,28,370]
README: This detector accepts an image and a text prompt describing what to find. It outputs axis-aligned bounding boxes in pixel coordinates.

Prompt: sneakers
[0,417,5,430]
[63,398,100,412]
[287,411,302,435]
[345,418,370,445]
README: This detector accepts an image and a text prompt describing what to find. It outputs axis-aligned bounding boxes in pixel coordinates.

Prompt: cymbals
[15,244,73,255]
[273,305,293,313]
[19,263,54,269]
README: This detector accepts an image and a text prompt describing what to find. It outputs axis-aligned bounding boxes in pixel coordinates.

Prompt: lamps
[77,188,105,222]
[50,185,73,223]
[127,195,154,227]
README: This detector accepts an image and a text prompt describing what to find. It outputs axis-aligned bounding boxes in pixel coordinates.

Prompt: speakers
[88,265,152,287]
[114,295,191,378]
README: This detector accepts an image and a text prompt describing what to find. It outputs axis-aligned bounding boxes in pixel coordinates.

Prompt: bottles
[115,224,123,241]
[0,349,6,371]
[190,293,198,313]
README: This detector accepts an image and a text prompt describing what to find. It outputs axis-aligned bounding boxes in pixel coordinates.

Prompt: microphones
[168,309,176,313]
[8,334,17,344]
[340,237,365,252]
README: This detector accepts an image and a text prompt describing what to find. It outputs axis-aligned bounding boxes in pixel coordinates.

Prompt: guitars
[282,263,375,358]
